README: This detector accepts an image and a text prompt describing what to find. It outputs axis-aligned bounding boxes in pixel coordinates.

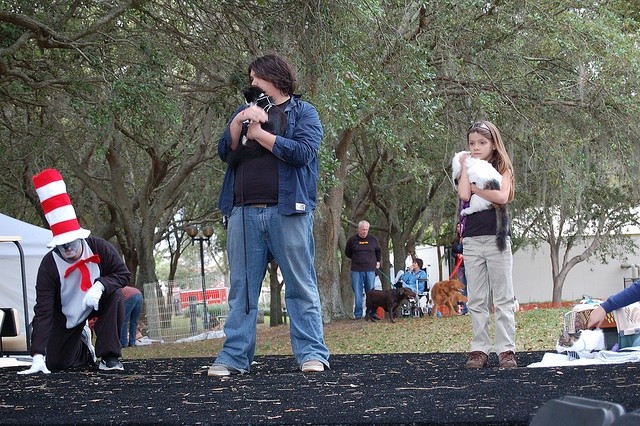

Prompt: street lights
[186,226,214,330]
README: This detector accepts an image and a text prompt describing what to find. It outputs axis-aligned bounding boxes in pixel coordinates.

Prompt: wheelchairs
[396,265,433,319]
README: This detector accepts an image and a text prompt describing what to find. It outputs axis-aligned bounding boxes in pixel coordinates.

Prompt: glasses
[469,121,489,130]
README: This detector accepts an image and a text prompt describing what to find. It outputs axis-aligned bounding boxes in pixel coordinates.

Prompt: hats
[32,168,92,248]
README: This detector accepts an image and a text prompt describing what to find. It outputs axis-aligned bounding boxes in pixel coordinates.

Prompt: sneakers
[83,327,97,363]
[207,363,230,377]
[301,360,324,372]
[99,357,124,372]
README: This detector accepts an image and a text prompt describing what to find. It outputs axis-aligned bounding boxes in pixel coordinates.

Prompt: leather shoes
[466,351,488,369]
[498,351,517,370]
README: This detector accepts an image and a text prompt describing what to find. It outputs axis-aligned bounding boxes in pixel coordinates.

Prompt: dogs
[432,279,467,319]
[366,286,416,324]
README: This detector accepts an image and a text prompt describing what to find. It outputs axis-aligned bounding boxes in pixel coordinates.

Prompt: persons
[207,52,331,377]
[451,221,468,315]
[17,224,133,375]
[385,258,427,319]
[455,120,519,369]
[587,277,640,330]
[346,220,383,323]
[121,286,144,349]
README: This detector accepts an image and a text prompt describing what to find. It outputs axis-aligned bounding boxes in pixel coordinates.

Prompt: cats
[556,328,606,360]
[451,150,509,253]
[226,87,288,170]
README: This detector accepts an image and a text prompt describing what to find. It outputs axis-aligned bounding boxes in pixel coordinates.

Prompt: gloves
[17,354,51,375]
[81,281,105,311]
